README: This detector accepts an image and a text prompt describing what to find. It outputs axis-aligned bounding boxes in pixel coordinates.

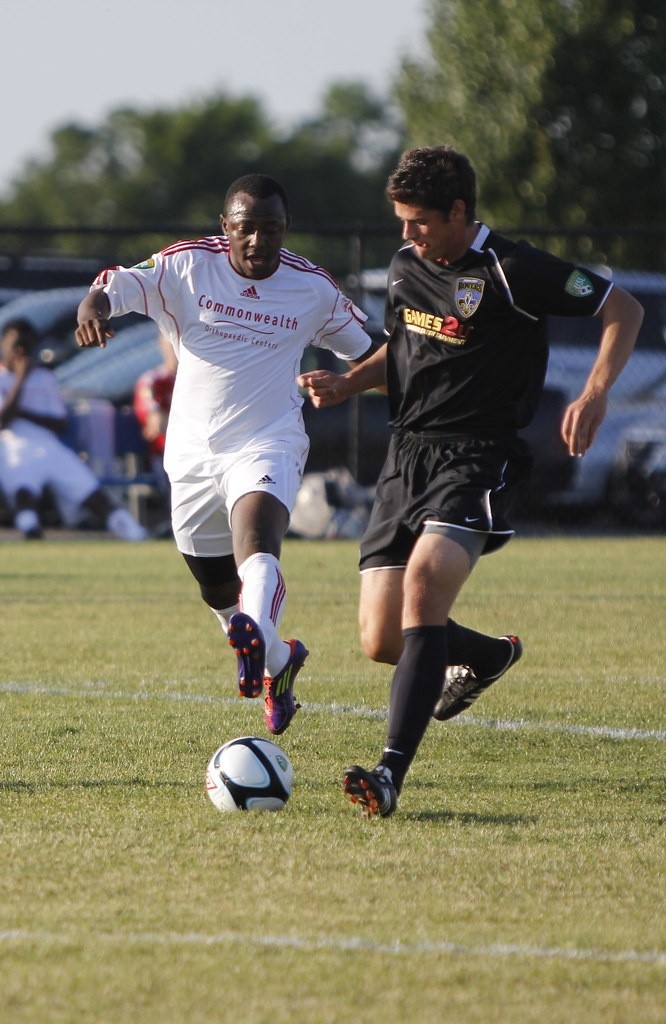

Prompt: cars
[0,272,666,511]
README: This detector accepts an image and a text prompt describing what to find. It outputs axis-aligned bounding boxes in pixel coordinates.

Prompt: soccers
[201,735,293,816]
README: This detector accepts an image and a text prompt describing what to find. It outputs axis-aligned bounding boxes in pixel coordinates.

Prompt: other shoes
[107,508,148,542]
[15,509,44,542]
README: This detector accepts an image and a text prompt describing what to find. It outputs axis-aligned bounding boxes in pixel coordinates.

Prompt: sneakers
[263,638,309,735]
[432,634,523,722]
[227,612,266,698]
[343,752,398,818]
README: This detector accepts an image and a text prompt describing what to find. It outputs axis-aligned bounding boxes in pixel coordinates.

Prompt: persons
[0,316,177,544]
[295,145,645,818]
[74,173,388,736]
